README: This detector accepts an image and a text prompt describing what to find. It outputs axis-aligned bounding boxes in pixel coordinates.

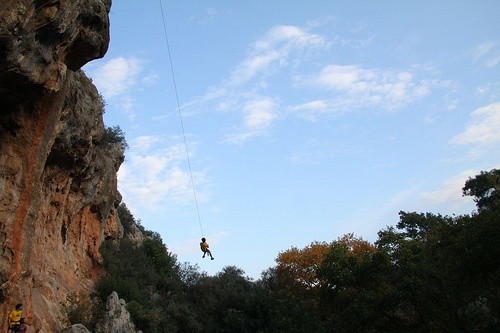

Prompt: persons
[200,238,214,260]
[18,316,26,333]
[7,303,23,333]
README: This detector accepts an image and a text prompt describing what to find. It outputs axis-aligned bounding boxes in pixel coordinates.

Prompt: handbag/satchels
[19,325,27,333]
[14,324,20,332]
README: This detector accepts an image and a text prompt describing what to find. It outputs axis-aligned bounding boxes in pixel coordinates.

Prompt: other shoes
[211,257,214,260]
[202,255,205,258]
